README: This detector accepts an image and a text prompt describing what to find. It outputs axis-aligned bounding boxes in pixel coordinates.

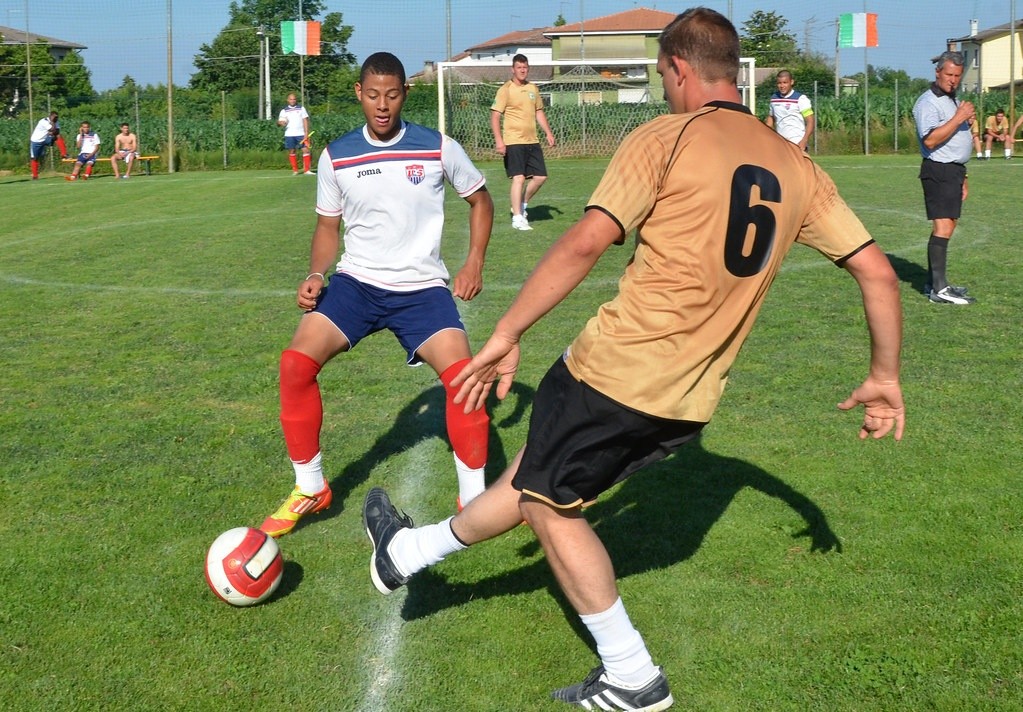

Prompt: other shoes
[984,156,990,161]
[977,155,983,161]
[1004,155,1012,160]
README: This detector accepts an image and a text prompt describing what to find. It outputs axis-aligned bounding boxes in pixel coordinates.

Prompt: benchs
[61,156,159,180]
[973,139,1023,142]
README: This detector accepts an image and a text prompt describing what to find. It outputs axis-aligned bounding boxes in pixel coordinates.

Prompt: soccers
[205,527,284,606]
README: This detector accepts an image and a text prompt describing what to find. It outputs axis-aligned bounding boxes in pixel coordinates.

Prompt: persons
[361,8,904,712]
[966,113,982,161]
[763,71,814,151]
[1009,114,1023,149]
[111,123,136,179]
[912,50,977,305]
[984,109,1013,161]
[258,53,529,538]
[65,121,101,181]
[30,111,69,181]
[278,94,317,176]
[491,54,555,231]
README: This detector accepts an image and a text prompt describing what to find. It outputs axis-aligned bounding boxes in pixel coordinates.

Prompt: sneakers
[114,174,130,180]
[509,206,528,219]
[61,154,72,159]
[304,171,316,176]
[81,174,88,181]
[929,286,976,305]
[923,283,970,297]
[361,485,414,596]
[512,215,534,230]
[552,661,673,712]
[260,476,333,540]
[65,174,79,181]
[293,171,299,176]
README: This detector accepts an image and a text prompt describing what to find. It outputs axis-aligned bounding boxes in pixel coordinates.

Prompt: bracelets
[305,272,325,282]
